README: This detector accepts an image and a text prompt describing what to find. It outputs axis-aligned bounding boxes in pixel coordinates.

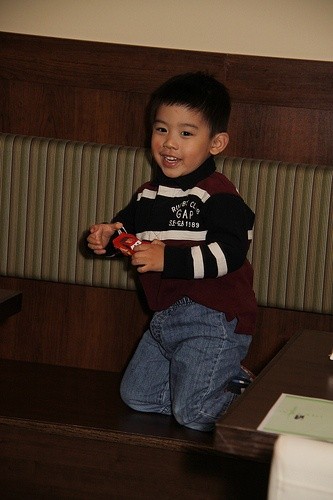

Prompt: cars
[112,232,145,257]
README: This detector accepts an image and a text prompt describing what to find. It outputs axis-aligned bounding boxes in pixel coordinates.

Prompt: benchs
[0,358,235,458]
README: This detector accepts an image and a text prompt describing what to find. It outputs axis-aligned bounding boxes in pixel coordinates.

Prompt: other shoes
[227,364,257,386]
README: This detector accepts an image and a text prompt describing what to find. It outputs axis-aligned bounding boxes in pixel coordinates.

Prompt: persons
[87,72,261,431]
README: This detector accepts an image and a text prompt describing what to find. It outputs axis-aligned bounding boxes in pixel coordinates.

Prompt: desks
[205,320,333,500]
